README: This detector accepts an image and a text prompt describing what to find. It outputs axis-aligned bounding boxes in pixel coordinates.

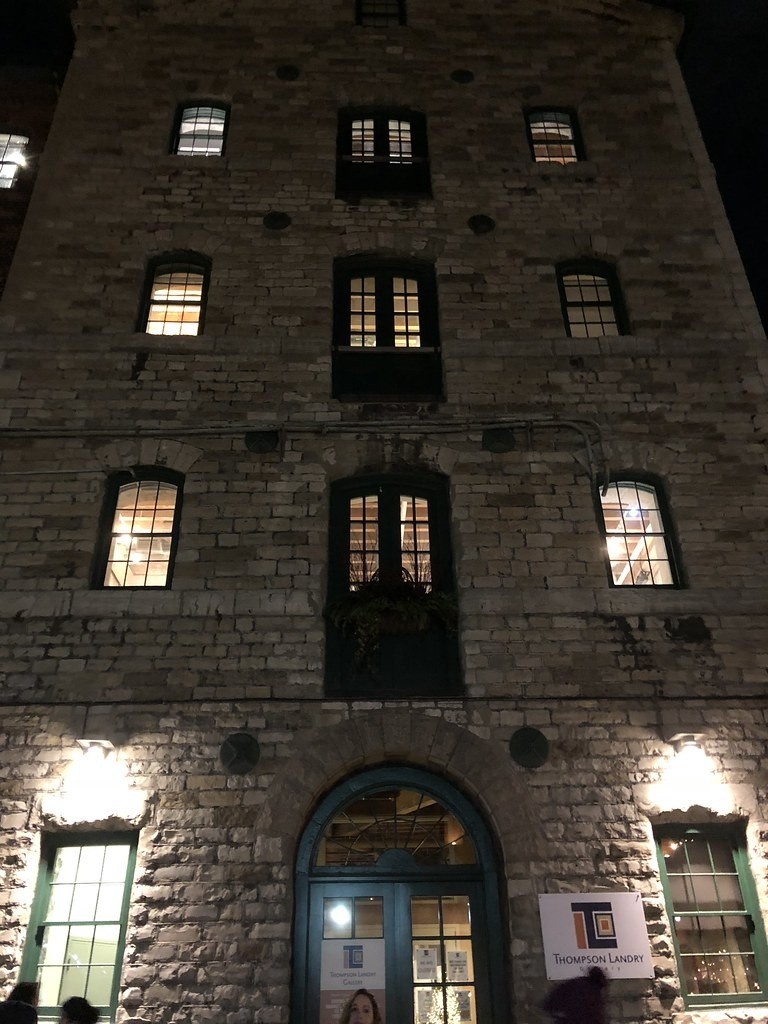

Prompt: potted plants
[321,544,461,640]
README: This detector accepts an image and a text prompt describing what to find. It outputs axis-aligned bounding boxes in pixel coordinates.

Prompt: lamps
[661,724,718,755]
[74,731,114,761]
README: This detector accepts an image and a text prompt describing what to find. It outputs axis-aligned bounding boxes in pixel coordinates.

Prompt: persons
[59,996,100,1024]
[0,982,39,1024]
[338,989,384,1024]
[539,966,611,1024]
